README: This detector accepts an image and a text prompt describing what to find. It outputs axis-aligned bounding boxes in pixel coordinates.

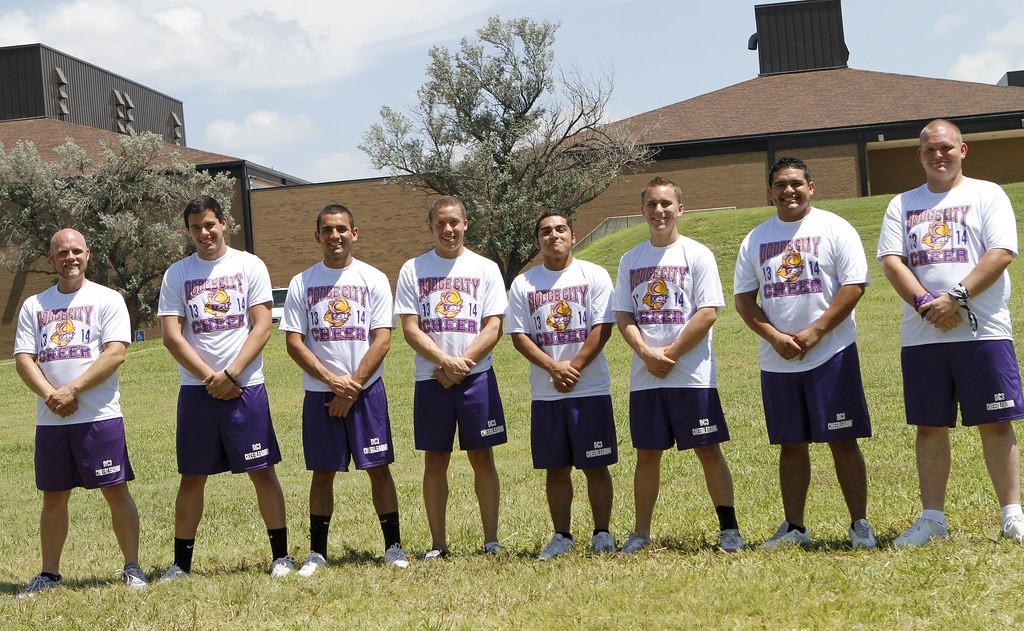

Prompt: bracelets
[224,369,236,384]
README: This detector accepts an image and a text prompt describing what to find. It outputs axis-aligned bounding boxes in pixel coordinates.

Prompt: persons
[614,177,743,554]
[11,229,154,597]
[151,194,296,585]
[506,211,617,563]
[279,204,413,577]
[733,157,879,554]
[878,120,1024,551]
[394,196,511,557]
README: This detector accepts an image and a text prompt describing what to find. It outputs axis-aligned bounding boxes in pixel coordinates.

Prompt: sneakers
[271,556,295,577]
[1002,513,1024,542]
[894,516,947,547]
[760,521,811,549]
[17,573,63,598]
[719,529,743,553]
[622,532,651,554]
[115,567,148,589]
[849,519,875,548]
[592,532,616,553]
[160,564,188,582]
[295,550,326,577]
[538,533,573,561]
[384,543,409,569]
[424,550,451,559]
[485,544,509,558]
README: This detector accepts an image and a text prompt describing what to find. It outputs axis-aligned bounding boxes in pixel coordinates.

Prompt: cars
[271,288,289,322]
